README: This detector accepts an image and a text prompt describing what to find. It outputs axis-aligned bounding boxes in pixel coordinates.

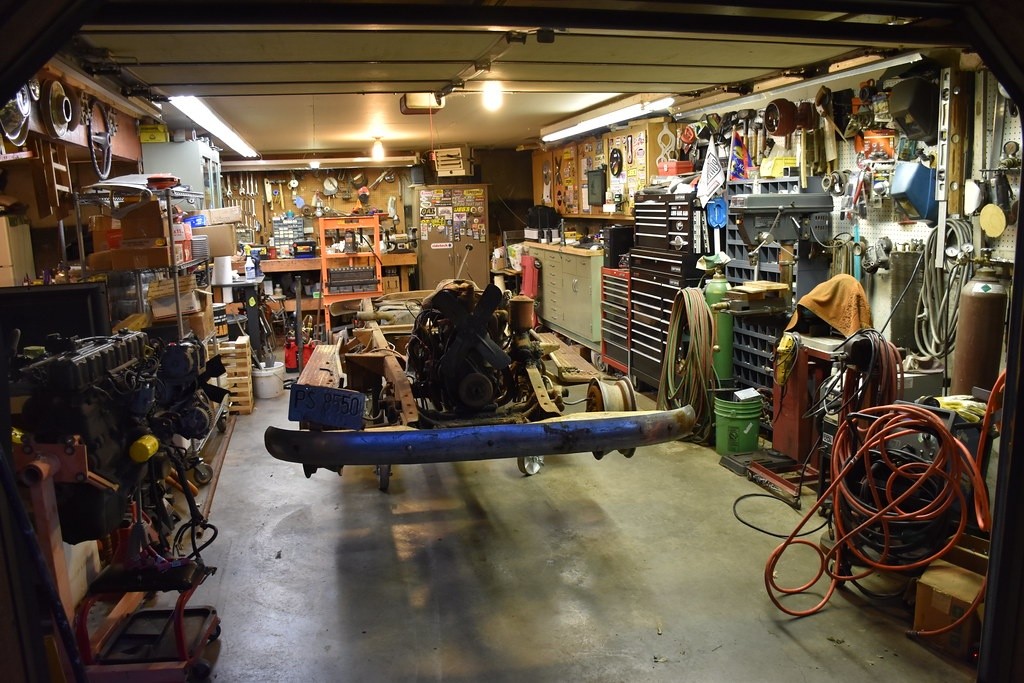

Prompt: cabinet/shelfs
[411,183,490,291]
[524,236,604,372]
[141,141,222,209]
[228,249,417,340]
[577,132,609,219]
[532,142,579,217]
[71,188,230,487]
[608,122,676,220]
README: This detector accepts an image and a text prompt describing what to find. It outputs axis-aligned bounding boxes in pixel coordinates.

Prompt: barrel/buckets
[252,361,284,398]
[713,389,764,457]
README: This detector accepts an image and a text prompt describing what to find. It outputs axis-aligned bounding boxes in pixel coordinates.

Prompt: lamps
[166,97,259,158]
[539,93,680,142]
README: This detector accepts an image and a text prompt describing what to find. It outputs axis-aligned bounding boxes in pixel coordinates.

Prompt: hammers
[759,110,767,159]
[748,123,762,164]
[737,109,757,180]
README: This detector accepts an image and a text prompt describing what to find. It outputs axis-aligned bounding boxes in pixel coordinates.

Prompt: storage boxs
[89,198,242,273]
[112,274,215,344]
[910,529,992,663]
[207,335,254,414]
